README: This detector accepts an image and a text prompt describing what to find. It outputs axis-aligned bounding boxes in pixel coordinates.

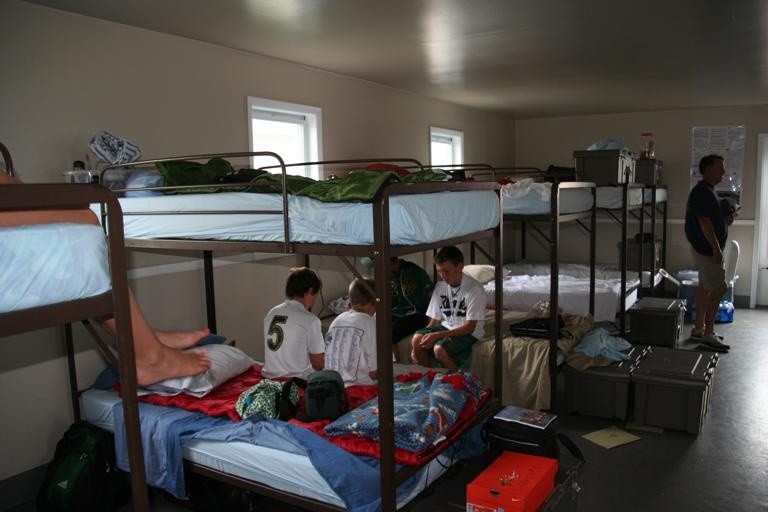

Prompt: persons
[0,168,210,386]
[261,266,327,382]
[409,245,485,371]
[685,154,734,352]
[364,255,433,364]
[322,276,378,387]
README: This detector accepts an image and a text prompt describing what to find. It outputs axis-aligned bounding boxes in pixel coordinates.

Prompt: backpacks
[37,420,116,512]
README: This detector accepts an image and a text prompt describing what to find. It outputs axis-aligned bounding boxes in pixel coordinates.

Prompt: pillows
[462,265,512,283]
[328,294,351,315]
[132,343,254,395]
[121,168,164,198]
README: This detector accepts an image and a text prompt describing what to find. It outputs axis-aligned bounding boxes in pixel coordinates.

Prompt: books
[581,425,641,450]
[494,404,557,430]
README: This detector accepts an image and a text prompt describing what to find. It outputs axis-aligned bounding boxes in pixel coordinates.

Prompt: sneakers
[700,334,730,351]
[690,326,724,342]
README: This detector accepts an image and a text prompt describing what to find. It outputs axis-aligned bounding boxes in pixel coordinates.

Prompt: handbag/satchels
[276,365,348,424]
[461,408,585,470]
[235,378,298,426]
[510,315,565,341]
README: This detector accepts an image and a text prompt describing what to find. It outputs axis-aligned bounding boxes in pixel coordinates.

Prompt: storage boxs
[633,346,720,436]
[466,451,558,512]
[563,346,651,421]
[408,454,584,512]
[628,295,688,350]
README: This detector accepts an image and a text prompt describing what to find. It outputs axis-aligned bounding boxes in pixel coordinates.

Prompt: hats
[361,257,377,268]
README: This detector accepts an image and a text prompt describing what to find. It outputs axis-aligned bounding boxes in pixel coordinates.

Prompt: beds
[455,167,642,337]
[632,184,667,297]
[2,146,668,511]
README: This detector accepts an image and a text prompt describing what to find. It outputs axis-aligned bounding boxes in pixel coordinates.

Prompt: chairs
[682,239,742,322]
